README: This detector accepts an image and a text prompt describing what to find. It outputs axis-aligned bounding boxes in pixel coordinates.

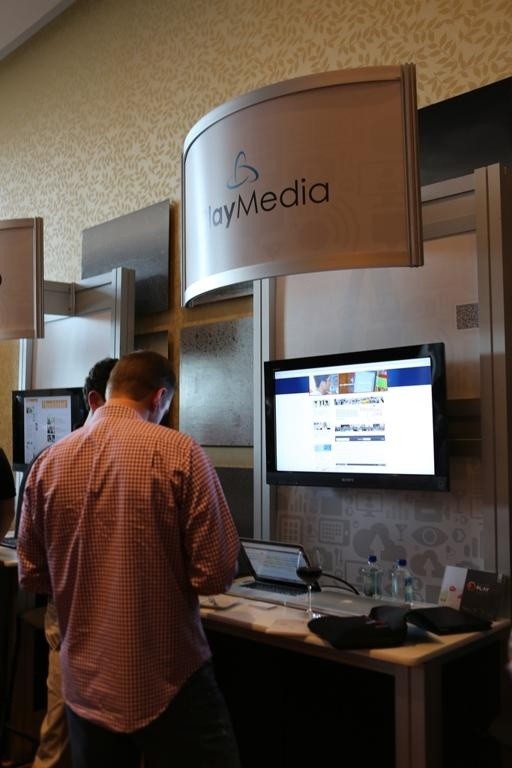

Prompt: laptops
[286,592,411,621]
[224,537,321,605]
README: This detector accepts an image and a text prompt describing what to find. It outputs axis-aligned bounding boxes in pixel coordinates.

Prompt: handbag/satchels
[307,605,411,650]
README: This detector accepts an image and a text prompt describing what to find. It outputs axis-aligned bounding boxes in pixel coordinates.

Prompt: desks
[196,575,512,768]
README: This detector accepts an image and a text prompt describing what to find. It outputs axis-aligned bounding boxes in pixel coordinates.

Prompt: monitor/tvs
[263,342,450,492]
[12,388,89,471]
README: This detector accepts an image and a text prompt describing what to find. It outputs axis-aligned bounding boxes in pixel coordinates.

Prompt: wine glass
[295,549,323,621]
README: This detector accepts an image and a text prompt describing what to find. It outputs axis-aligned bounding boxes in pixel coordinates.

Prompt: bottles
[361,553,382,598]
[390,559,411,601]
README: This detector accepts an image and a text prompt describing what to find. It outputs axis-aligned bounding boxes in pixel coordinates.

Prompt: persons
[16,351,244,768]
[32,356,117,768]
[0,448,17,542]
